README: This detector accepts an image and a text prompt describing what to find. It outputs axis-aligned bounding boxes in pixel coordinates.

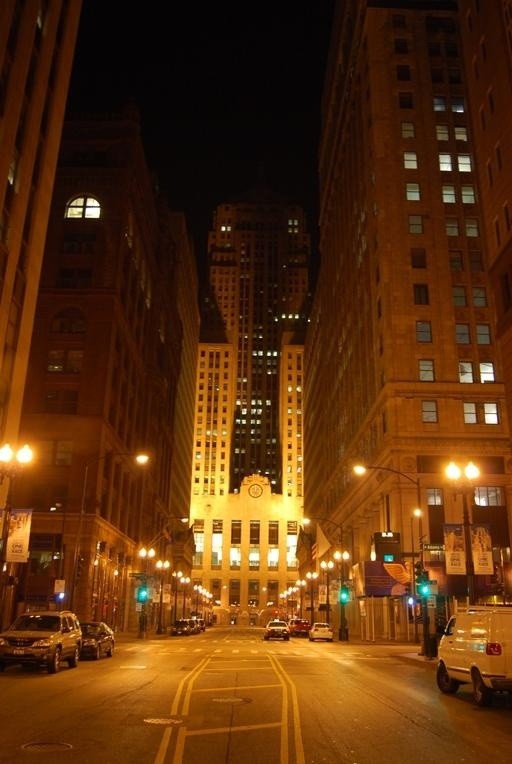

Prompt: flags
[295,522,333,578]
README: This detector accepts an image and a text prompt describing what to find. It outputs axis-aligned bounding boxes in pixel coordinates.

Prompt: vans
[436,608,510,705]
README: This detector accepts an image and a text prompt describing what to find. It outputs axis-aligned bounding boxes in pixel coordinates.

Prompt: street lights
[354,463,425,562]
[279,559,333,640]
[70,451,149,610]
[155,560,212,640]
[446,462,479,604]
[301,517,350,641]
[1,442,34,574]
[137,548,154,637]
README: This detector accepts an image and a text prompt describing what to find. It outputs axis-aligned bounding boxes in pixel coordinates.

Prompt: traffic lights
[340,588,350,602]
[136,588,147,602]
[414,562,433,595]
[408,597,414,605]
[58,592,65,599]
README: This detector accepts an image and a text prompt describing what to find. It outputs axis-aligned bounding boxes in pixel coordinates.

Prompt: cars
[174,619,205,635]
[287,619,310,636]
[309,623,334,642]
[265,619,289,641]
[0,610,114,673]
